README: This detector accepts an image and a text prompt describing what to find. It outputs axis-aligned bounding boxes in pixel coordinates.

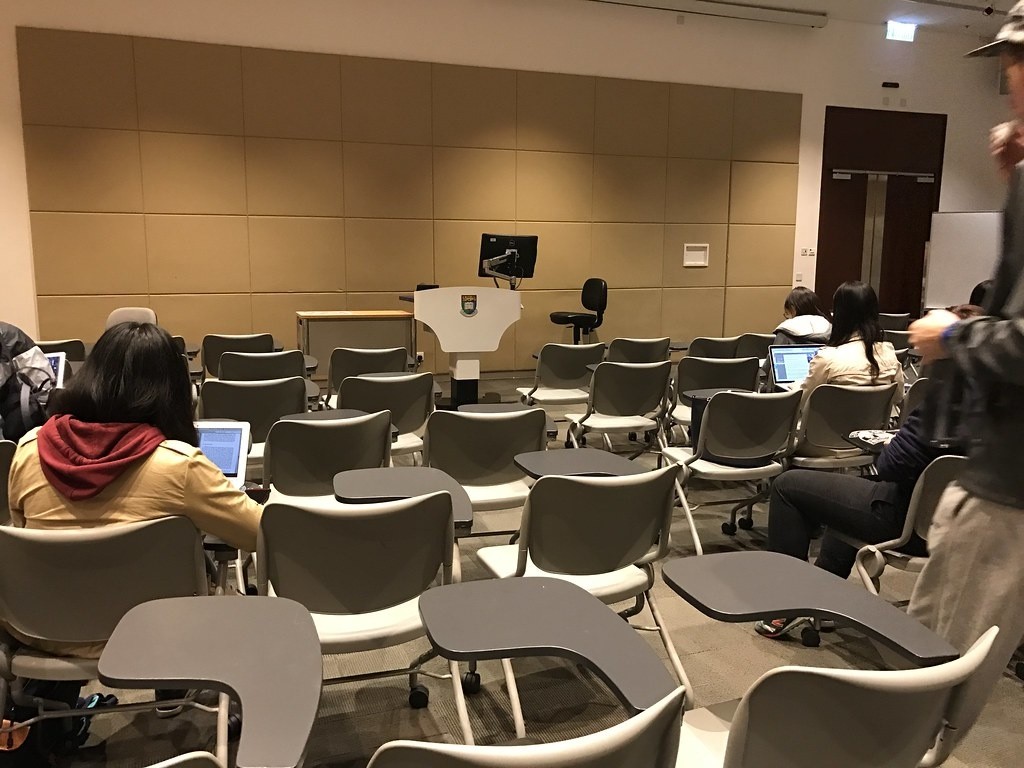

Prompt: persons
[743,279,992,639]
[0,322,56,445]
[907,0,1024,768]
[8,319,267,755]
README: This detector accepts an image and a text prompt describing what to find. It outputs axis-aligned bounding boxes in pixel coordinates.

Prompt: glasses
[783,311,793,319]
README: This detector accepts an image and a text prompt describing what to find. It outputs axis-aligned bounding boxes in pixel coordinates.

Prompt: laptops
[193,421,251,493]
[768,344,826,391]
[44,352,66,389]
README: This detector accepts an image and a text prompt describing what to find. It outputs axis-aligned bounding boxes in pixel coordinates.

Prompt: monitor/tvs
[477,233,538,279]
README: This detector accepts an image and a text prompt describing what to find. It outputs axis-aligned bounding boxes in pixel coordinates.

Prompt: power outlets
[417,352,424,361]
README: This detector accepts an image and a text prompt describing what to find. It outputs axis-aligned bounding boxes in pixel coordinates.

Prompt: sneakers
[153,688,193,717]
[753,617,798,636]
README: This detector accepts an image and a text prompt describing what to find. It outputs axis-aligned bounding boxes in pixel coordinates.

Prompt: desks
[295,310,415,380]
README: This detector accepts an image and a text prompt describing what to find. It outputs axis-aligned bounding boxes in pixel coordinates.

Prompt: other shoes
[1014,661,1024,680]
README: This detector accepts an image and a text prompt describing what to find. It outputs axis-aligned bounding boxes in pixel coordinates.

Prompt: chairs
[0,306,1024,767]
[550,278,607,345]
